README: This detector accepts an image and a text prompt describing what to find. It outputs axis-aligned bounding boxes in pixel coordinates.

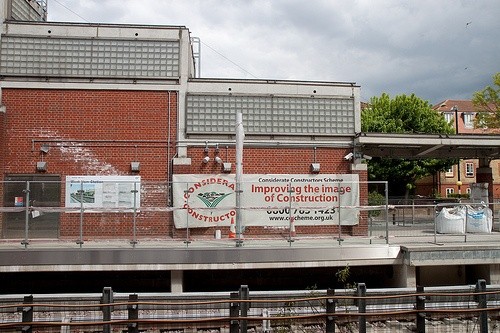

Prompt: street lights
[450,104,463,193]
[456,181,463,203]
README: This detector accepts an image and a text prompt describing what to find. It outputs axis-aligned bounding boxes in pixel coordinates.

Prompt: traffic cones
[229,217,236,238]
[289,217,296,237]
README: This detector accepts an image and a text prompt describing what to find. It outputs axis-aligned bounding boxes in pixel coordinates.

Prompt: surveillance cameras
[363,154,372,160]
[204,147,220,154]
[344,152,353,160]
